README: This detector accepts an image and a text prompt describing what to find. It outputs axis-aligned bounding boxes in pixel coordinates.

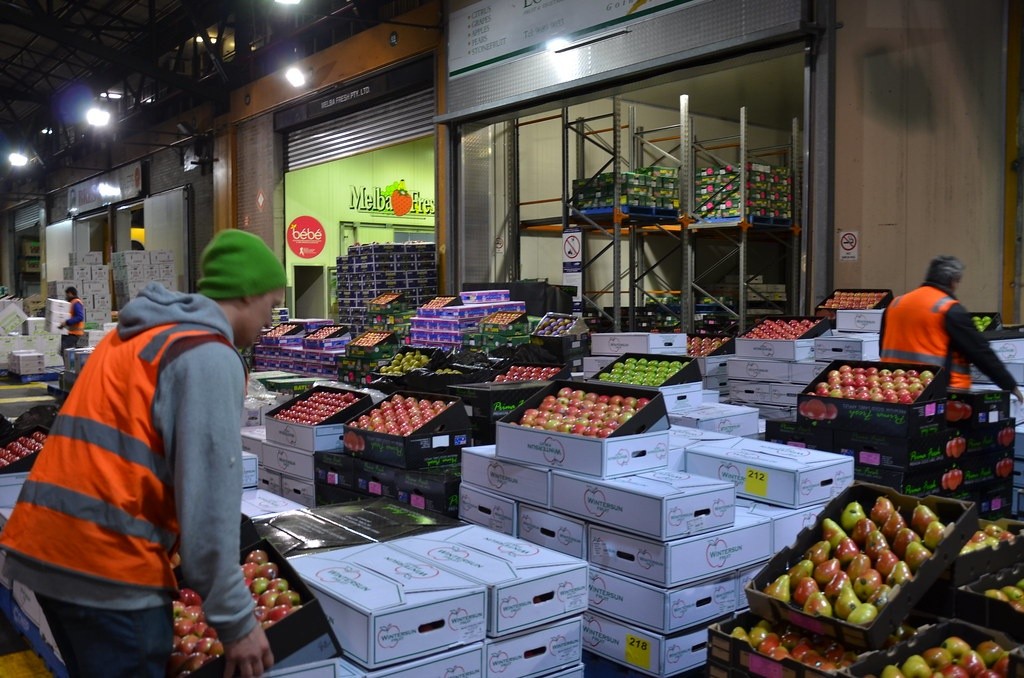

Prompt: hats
[925,254,966,281]
[196,228,287,299]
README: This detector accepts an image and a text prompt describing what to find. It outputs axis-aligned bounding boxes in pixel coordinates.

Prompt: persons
[57,286,85,349]
[879,256,1023,403]
[0,229,287,678]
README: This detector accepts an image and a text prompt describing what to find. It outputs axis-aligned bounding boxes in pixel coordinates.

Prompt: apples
[170,549,301,678]
[263,292,518,438]
[506,289,991,440]
[0,431,47,469]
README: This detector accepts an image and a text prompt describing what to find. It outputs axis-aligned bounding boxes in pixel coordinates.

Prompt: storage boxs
[0,163,1024,677]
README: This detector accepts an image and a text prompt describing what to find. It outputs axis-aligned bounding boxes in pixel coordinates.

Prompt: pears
[724,496,1024,678]
